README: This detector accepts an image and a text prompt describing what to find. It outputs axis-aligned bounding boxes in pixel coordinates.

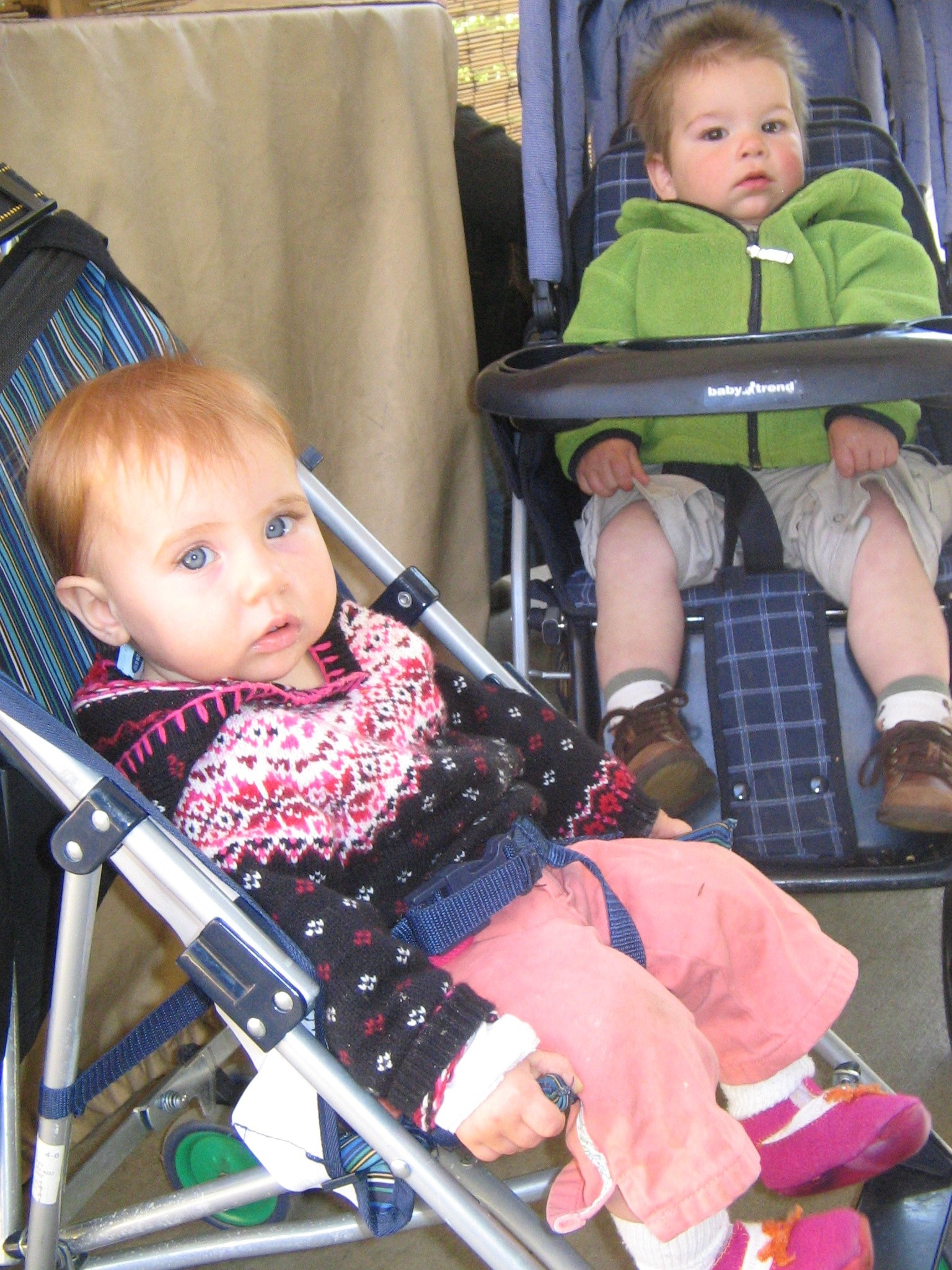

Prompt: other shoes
[744,1080,932,1196]
[711,1207,876,1270]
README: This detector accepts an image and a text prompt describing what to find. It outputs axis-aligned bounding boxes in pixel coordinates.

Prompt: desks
[0,7,490,643]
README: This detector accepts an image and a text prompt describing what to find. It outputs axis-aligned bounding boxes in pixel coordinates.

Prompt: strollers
[469,0,948,1017]
[0,160,951,1269]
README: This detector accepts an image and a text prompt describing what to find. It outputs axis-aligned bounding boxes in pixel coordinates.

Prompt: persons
[27,356,933,1270]
[558,0,952,834]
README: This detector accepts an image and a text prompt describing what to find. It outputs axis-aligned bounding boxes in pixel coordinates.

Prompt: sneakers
[859,718,952,836]
[597,688,716,816]
